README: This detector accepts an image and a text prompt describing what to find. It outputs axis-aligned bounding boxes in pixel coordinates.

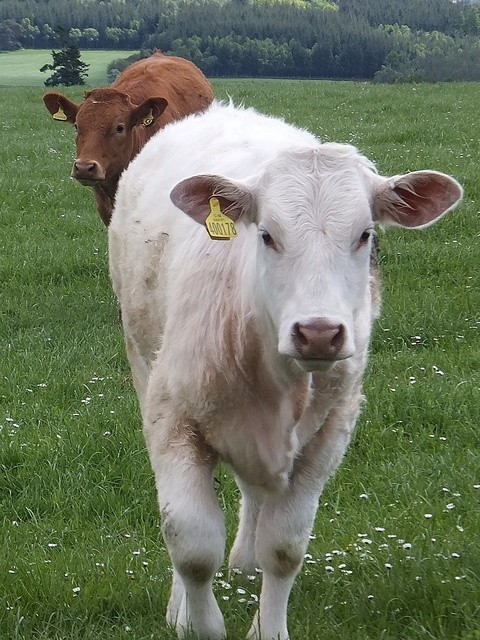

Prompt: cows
[106,92,465,640]
[42,52,214,226]
